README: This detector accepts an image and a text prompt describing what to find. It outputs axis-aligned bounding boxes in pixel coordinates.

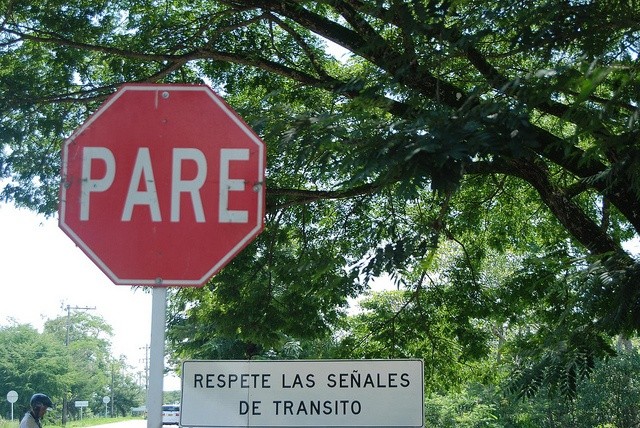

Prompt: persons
[19,393,53,428]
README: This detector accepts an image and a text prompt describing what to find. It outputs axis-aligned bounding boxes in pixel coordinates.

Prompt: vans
[161,405,180,425]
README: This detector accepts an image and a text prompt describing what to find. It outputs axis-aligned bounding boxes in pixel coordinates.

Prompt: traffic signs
[182,360,422,425]
